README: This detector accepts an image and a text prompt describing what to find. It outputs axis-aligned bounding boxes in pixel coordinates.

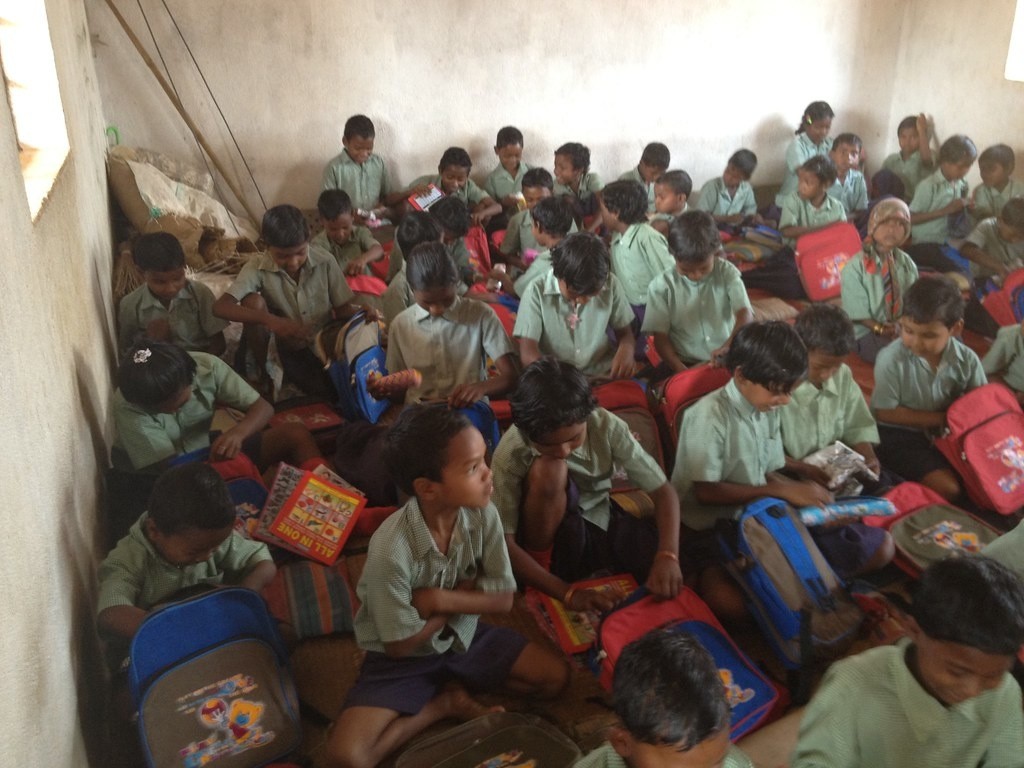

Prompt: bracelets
[872,322,884,338]
[656,550,676,560]
[563,585,577,604]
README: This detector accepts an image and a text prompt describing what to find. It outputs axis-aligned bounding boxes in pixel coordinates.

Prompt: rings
[219,445,226,450]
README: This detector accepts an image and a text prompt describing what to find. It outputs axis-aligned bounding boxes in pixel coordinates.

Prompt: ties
[876,246,901,320]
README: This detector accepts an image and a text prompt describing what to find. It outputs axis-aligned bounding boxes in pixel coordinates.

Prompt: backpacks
[591,375,668,520]
[305,312,395,424]
[394,710,584,768]
[263,392,350,442]
[714,501,864,667]
[463,226,494,287]
[165,442,294,566]
[860,481,1003,587]
[401,397,500,465]
[973,266,1024,334]
[345,273,389,316]
[114,584,300,768]
[924,382,1024,515]
[582,581,782,746]
[736,219,788,253]
[794,221,864,301]
[649,361,732,451]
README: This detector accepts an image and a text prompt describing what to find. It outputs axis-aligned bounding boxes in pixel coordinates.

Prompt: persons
[791,557,1024,768]
[570,629,757,768]
[95,100,1024,646]
[490,358,684,616]
[325,406,567,768]
